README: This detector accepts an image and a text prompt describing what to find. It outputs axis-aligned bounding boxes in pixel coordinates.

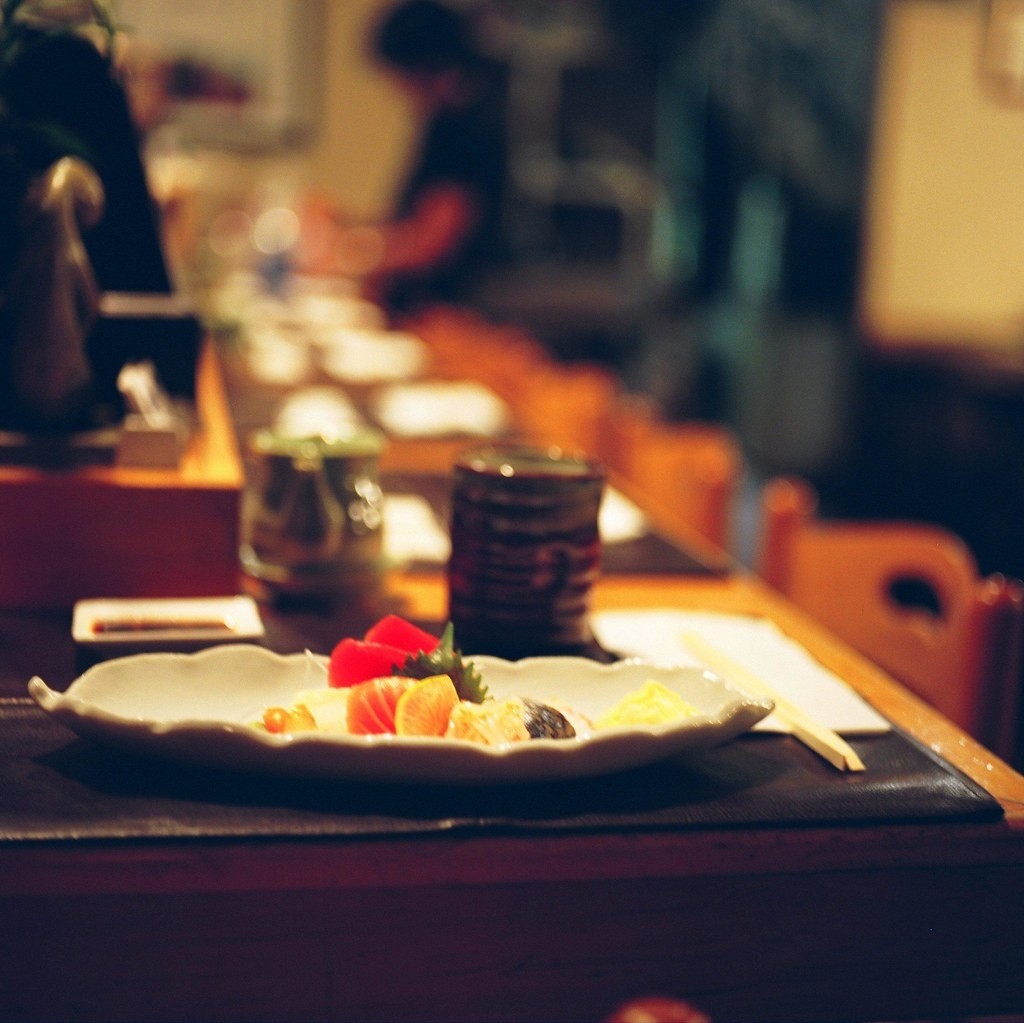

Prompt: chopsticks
[684,633,865,772]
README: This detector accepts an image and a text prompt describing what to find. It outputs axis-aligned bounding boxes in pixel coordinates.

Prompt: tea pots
[238,424,386,612]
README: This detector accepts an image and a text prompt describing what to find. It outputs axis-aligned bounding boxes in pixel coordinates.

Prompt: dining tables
[0,329,1024,1023]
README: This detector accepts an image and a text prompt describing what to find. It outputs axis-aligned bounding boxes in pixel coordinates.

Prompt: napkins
[377,378,511,440]
[324,335,429,382]
[590,603,893,735]
[599,485,646,544]
[296,295,378,325]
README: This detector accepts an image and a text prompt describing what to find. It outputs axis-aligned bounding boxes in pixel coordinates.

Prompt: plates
[27,643,776,786]
[70,596,263,664]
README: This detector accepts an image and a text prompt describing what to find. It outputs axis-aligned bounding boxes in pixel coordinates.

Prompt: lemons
[394,673,460,738]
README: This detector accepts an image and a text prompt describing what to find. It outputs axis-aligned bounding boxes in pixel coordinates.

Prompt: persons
[0,1,206,435]
[285,0,512,327]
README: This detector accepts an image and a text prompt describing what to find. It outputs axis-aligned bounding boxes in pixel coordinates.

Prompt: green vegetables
[393,621,494,703]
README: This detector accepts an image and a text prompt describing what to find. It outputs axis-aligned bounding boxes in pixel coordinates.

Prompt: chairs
[758,476,1024,739]
[414,306,741,547]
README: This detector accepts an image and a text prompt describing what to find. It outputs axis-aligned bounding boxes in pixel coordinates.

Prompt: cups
[447,443,607,647]
